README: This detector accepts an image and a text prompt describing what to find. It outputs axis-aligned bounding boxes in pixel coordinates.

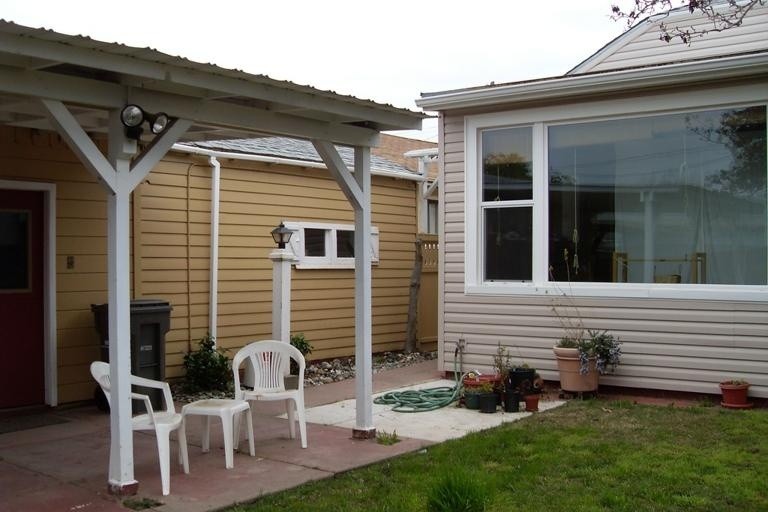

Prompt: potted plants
[460,367,546,416]
[716,378,751,409]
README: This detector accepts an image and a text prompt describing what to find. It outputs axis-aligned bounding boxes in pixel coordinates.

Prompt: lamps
[116,102,172,143]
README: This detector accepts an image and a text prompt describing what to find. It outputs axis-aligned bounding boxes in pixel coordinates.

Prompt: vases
[552,344,600,400]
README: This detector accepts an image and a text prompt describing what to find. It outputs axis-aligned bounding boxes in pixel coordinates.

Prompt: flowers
[546,331,623,376]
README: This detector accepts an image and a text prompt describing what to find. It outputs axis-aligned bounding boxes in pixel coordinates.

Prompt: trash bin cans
[90,298,174,414]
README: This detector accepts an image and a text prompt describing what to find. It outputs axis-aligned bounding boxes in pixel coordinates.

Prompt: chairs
[88,340,309,493]
